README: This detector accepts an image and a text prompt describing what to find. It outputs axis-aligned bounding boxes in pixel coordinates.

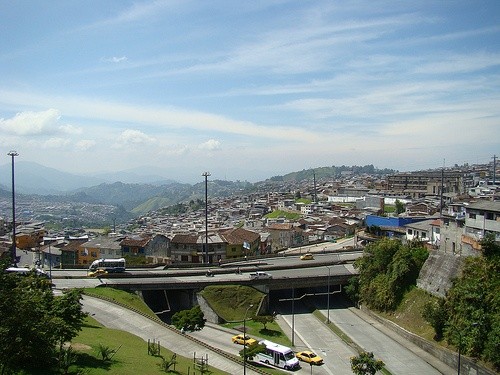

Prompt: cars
[300,253,314,260]
[87,269,109,279]
[231,333,256,347]
[295,350,324,365]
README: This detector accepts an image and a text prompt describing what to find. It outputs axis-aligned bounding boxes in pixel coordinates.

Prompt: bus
[256,339,300,371]
[4,267,51,279]
[88,257,127,273]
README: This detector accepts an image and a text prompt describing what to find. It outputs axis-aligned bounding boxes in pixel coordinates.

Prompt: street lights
[7,150,20,267]
[201,171,212,263]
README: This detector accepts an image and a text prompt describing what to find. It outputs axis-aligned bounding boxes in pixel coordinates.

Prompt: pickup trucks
[248,272,273,280]
